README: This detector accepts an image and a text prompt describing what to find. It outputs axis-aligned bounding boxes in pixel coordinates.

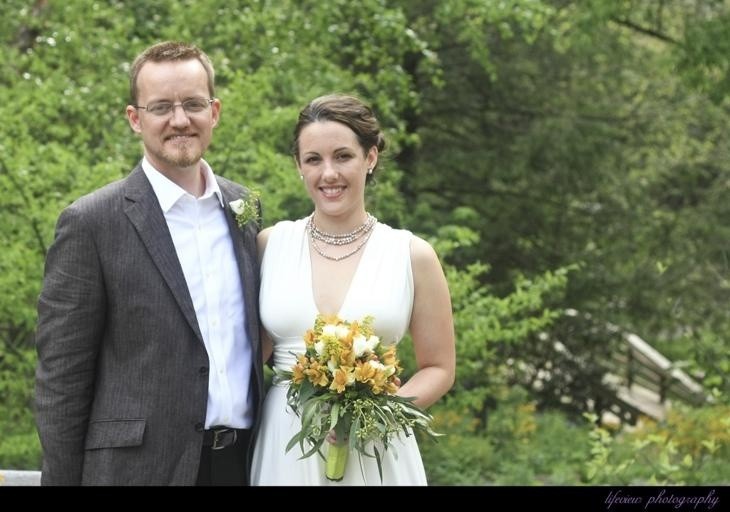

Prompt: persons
[249,92,457,487]
[33,39,265,488]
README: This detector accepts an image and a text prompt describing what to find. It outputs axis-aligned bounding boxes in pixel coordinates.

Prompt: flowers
[284,314,448,484]
[228,184,266,230]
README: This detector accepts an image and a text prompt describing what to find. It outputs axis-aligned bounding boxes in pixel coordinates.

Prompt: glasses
[132,98,215,115]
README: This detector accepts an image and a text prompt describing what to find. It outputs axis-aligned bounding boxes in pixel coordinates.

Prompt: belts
[203,427,253,450]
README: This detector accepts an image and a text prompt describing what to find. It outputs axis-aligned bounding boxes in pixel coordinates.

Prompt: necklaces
[304,210,377,261]
